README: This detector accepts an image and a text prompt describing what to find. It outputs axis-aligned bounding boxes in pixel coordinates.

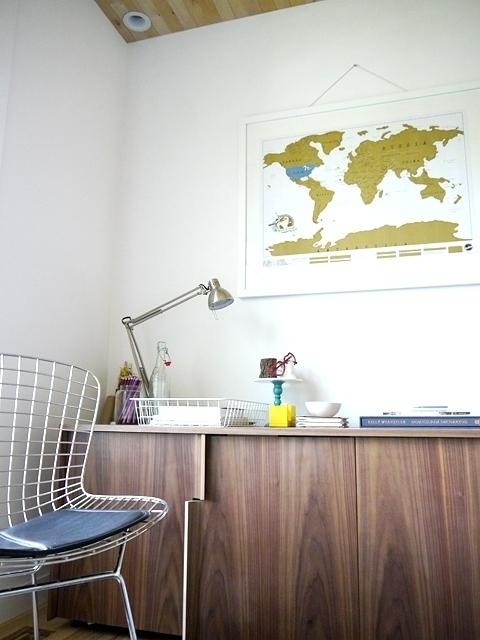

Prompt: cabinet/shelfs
[48,420,358,640]
[356,435,480,640]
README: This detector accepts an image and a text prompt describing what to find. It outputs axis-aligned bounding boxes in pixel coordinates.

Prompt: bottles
[148,341,172,406]
[114,384,142,425]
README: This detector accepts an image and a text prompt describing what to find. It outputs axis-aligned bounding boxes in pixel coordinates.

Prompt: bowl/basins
[303,401,341,417]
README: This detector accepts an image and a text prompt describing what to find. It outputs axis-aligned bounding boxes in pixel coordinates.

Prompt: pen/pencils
[118,376,141,424]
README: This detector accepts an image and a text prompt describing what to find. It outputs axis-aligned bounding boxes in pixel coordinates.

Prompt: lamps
[121,278,234,392]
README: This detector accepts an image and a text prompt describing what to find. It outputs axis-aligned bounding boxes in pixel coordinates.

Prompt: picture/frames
[235,79,480,297]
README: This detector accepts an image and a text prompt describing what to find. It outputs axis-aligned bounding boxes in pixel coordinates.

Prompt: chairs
[0,351,169,640]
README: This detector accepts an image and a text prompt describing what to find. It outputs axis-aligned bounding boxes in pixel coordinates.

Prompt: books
[154,406,248,426]
[294,416,349,428]
[360,417,480,428]
